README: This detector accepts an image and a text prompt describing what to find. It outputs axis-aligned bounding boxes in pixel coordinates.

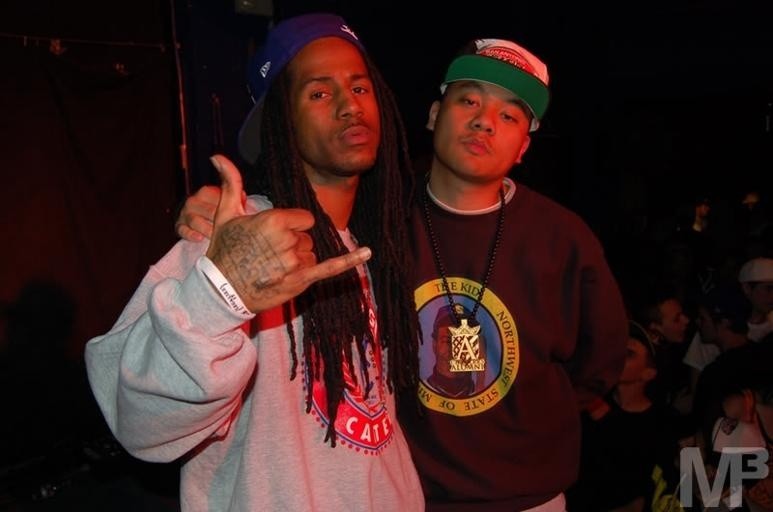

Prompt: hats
[441,39,550,122]
[237,13,366,165]
